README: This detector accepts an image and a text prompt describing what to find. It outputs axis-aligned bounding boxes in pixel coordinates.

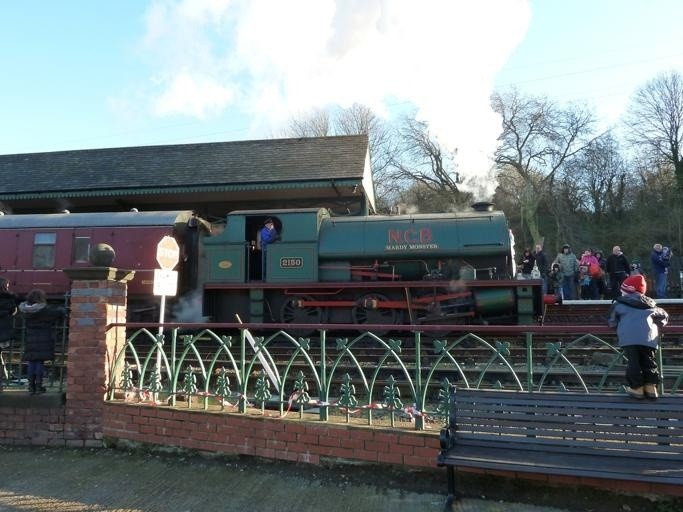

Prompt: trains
[0,200,554,364]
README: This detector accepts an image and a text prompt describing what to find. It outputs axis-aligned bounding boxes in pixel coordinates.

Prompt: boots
[35,383,48,394]
[27,380,35,394]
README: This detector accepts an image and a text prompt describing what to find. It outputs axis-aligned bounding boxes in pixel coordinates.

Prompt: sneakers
[619,383,645,399]
[643,382,659,400]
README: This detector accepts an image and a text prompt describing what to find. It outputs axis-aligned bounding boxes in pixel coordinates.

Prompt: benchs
[433,382,681,511]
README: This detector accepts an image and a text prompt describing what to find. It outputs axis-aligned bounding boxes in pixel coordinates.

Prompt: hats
[553,265,560,273]
[620,273,647,296]
[561,244,571,254]
[264,217,273,224]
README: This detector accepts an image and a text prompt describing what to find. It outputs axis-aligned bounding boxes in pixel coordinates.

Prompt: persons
[660,246,673,274]
[650,244,671,299]
[516,243,630,300]
[608,273,670,399]
[19,289,65,394]
[0,277,18,392]
[261,218,278,245]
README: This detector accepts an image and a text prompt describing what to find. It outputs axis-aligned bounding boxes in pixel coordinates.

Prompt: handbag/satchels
[589,262,602,279]
